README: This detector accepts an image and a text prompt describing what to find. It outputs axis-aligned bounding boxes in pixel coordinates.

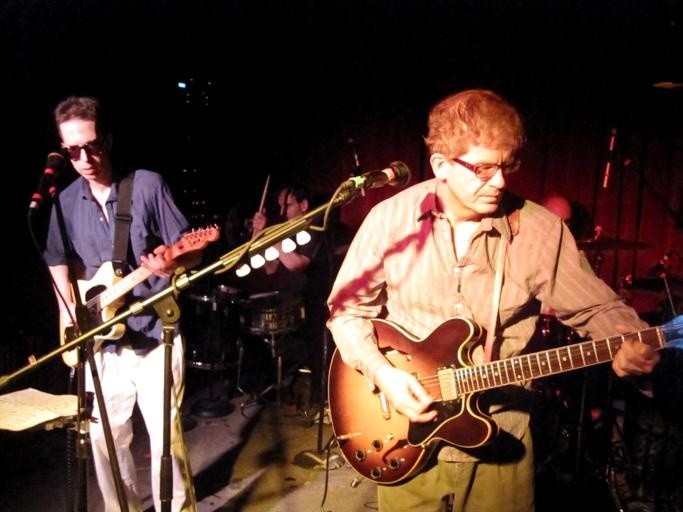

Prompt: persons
[223,200,251,244]
[325,91,658,512]
[243,181,330,400]
[41,96,203,512]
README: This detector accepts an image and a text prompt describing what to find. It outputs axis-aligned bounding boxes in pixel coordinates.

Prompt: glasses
[62,138,104,160]
[453,158,522,180]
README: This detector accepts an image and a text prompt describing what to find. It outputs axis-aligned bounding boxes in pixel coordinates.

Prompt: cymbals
[575,237,646,251]
[625,278,680,290]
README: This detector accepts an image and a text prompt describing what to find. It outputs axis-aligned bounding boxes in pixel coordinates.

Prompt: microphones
[595,115,627,198]
[29,149,70,212]
[337,160,411,204]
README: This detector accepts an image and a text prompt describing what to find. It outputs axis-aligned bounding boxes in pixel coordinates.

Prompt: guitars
[328,316,683,485]
[60,223,219,369]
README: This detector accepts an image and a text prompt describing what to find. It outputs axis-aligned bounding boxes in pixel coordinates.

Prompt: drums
[217,285,279,303]
[238,295,304,334]
[183,294,236,368]
